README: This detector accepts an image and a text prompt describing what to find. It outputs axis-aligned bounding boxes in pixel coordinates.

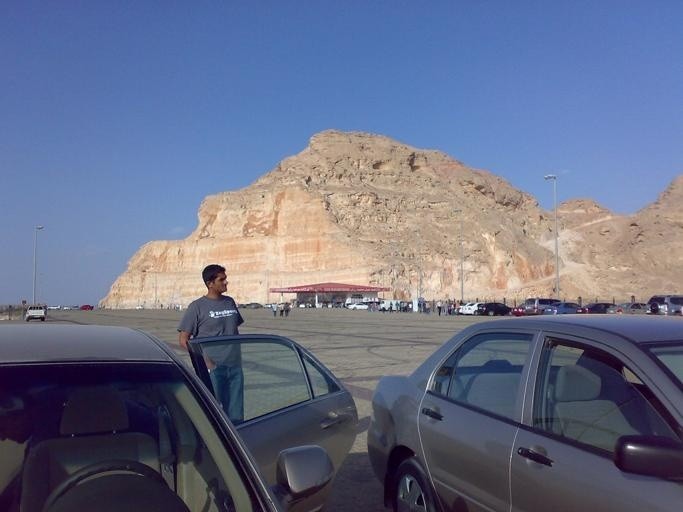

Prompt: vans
[525,298,562,314]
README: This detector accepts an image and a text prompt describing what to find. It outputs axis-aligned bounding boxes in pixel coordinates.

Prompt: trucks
[380,300,402,311]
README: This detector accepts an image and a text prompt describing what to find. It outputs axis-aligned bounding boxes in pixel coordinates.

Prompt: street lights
[544,174,559,300]
[32,225,45,305]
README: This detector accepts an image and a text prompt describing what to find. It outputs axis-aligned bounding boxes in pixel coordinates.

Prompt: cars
[47,304,94,311]
[238,302,369,310]
[366,313,683,511]
[458,303,512,315]
[544,301,581,314]
[511,303,525,315]
[604,303,646,314]
[135,305,144,310]
[0,323,362,512]
[575,303,616,314]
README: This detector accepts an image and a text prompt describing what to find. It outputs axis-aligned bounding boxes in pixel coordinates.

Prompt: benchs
[457,373,555,428]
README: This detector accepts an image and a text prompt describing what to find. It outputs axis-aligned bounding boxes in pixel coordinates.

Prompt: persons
[175,264,244,422]
[271,295,541,319]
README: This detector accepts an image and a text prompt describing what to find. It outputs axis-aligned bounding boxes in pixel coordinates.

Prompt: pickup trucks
[25,306,45,321]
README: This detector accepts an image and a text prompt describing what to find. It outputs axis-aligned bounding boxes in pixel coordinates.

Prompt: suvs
[646,295,683,314]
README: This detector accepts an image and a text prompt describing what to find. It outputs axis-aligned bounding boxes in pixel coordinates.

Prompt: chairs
[551,360,643,454]
[21,393,166,512]
